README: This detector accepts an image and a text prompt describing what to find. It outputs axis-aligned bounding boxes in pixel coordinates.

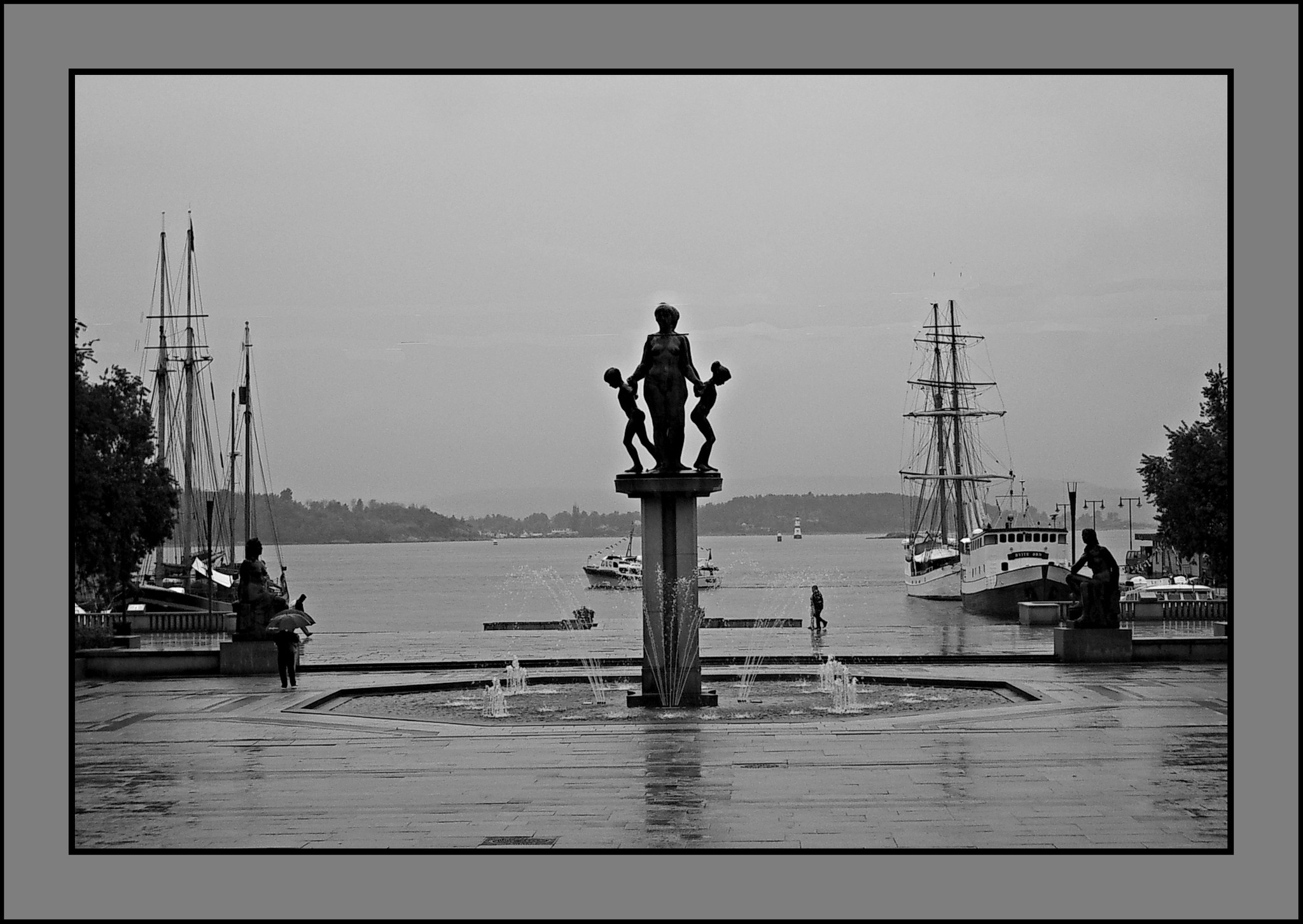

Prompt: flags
[1121,546,1163,574]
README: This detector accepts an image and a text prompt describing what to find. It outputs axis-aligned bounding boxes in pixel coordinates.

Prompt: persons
[1066,528,1119,628]
[604,367,656,472]
[808,585,828,630]
[627,304,704,469]
[237,537,288,641]
[690,361,732,472]
[295,594,313,637]
[273,630,300,690]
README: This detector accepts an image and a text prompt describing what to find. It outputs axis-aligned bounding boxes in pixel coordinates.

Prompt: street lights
[1082,499,1106,531]
[1117,496,1143,549]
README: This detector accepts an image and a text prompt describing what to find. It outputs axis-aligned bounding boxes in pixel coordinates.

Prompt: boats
[493,540,498,545]
[958,476,1093,618]
[582,521,722,589]
[1119,575,1214,620]
[519,530,544,538]
[545,527,579,538]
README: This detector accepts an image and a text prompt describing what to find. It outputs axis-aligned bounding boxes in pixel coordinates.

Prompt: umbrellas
[266,608,316,632]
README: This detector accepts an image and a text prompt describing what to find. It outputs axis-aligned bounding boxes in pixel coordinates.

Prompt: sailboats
[121,209,291,619]
[898,298,1018,602]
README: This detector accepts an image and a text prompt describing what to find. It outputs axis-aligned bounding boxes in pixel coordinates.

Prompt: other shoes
[808,626,815,630]
[823,621,828,627]
[815,627,821,629]
[281,687,286,690]
[291,685,297,689]
